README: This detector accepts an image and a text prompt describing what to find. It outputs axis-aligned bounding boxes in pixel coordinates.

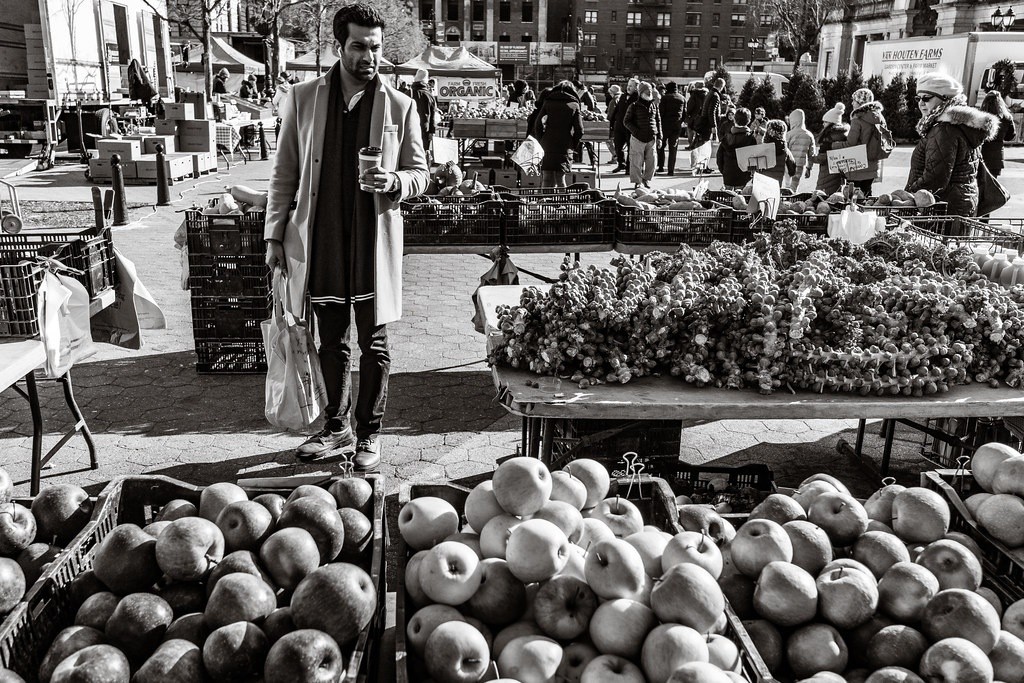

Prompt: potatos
[462,107,606,121]
[519,187,935,236]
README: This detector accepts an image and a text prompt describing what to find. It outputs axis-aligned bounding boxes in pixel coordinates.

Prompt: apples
[676,440,1024,683]
[397,455,750,683]
[0,469,378,683]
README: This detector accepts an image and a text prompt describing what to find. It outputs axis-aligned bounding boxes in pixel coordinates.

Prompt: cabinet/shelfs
[0,99,58,171]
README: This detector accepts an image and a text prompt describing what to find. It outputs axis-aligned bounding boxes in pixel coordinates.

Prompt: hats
[916,71,963,100]
[637,81,651,96]
[608,85,624,94]
[822,102,846,126]
[851,88,874,105]
[513,80,527,93]
[413,68,429,85]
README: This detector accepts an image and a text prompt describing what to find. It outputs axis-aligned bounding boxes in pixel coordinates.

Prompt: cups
[358,145,382,191]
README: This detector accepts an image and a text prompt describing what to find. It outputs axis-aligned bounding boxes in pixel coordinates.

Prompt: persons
[399,67,435,150]
[266,3,431,472]
[786,108,815,192]
[904,71,999,235]
[811,102,850,196]
[759,120,795,188]
[716,107,757,189]
[213,67,299,141]
[979,90,1016,223]
[509,78,769,190]
[832,88,886,196]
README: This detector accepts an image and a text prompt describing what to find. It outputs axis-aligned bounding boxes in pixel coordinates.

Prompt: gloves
[822,141,832,150]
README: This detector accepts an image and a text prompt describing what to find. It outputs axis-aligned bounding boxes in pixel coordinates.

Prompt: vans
[703,71,791,100]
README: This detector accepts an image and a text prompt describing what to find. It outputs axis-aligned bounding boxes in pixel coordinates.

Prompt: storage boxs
[89,92,218,179]
[186,206,274,374]
[0,418,1024,683]
[240,124,260,147]
[0,227,119,337]
[453,117,611,188]
[15,23,49,99]
[401,187,948,245]
[0,90,25,99]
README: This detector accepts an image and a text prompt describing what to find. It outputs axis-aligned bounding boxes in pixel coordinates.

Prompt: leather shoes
[295,422,354,457]
[353,430,381,470]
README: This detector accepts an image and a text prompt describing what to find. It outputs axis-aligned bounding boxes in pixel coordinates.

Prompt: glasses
[915,95,937,102]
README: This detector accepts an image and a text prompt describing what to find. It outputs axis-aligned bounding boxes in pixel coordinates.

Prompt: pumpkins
[201,184,267,247]
[402,162,491,233]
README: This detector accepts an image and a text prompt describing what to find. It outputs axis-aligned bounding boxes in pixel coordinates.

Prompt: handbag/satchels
[977,159,1010,217]
[260,264,329,430]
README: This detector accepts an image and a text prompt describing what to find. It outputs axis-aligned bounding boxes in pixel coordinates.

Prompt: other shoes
[608,152,717,190]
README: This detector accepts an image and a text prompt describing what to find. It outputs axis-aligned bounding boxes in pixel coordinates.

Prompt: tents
[288,40,395,89]
[173,35,265,93]
[396,46,502,99]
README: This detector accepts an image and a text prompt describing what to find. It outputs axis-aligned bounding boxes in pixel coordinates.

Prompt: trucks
[861,31,1023,146]
[0,0,190,159]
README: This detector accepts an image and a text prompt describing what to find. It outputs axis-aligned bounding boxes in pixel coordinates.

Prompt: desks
[216,123,246,169]
[401,245,707,282]
[223,119,276,160]
[0,289,115,498]
[451,136,609,190]
[477,283,1023,465]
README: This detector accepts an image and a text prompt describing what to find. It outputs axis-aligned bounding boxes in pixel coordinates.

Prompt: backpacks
[866,122,896,160]
